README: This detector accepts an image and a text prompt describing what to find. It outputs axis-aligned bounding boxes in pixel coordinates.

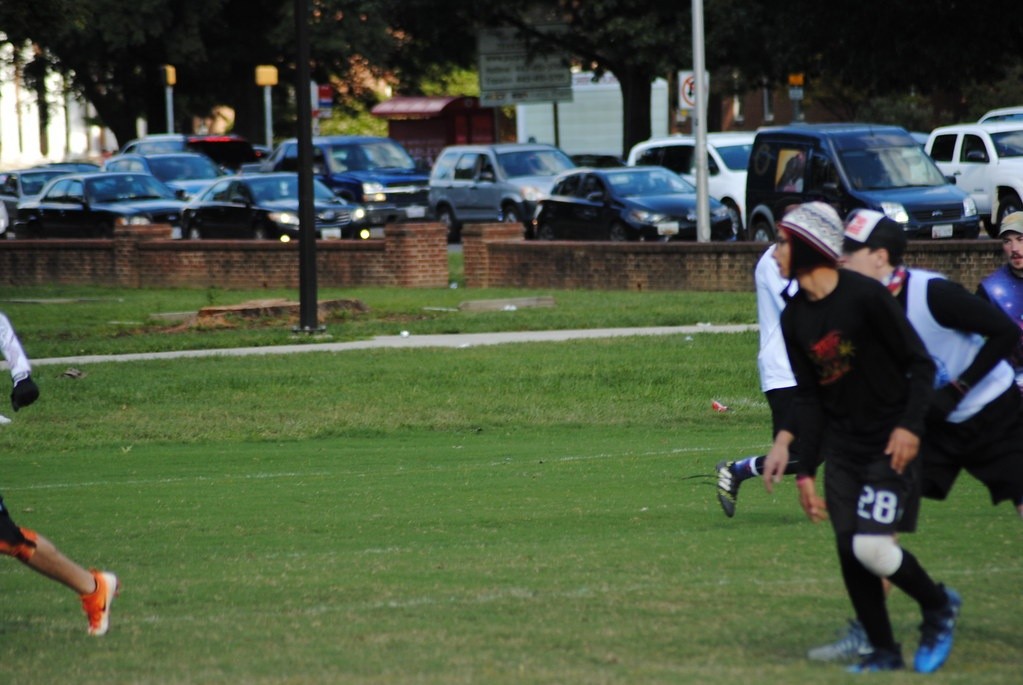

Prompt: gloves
[11,378,39,412]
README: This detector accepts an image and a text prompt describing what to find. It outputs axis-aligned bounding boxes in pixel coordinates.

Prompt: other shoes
[808,619,874,661]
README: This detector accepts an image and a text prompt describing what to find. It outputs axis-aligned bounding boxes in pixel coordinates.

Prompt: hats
[779,201,844,263]
[844,208,907,256]
[998,211,1023,234]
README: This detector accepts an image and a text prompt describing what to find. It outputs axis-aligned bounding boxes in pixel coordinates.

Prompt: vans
[745,121,981,241]
[117,134,261,175]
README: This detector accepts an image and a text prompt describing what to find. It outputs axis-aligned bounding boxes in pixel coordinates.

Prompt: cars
[427,142,581,244]
[179,171,374,241]
[13,171,191,240]
[531,165,736,242]
[261,133,433,229]
[910,105,1023,239]
[627,129,758,241]
[0,160,105,238]
[99,152,237,204]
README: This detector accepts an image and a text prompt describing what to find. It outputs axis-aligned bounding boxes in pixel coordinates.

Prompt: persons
[563,177,578,196]
[179,163,196,179]
[0,312,120,637]
[807,208,1023,664]
[977,210,1023,388]
[778,152,806,192]
[597,177,625,201]
[714,203,832,525]
[824,163,862,189]
[116,177,133,195]
[770,203,963,674]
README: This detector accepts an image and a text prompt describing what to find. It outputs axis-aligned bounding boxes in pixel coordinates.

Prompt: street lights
[161,65,176,134]
[255,65,279,152]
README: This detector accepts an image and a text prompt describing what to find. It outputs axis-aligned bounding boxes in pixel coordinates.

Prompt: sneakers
[914,582,960,674]
[846,644,903,673]
[82,567,117,637]
[717,460,741,518]
